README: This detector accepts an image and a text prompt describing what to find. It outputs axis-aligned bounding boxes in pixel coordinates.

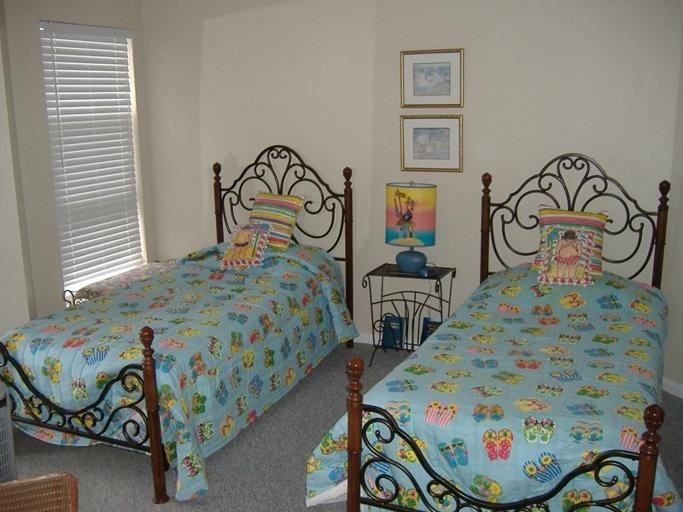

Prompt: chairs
[0,473,78,512]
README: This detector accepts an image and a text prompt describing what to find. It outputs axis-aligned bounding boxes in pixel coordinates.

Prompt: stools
[61,259,175,307]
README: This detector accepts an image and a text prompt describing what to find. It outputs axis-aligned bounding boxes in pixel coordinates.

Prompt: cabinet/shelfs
[362,262,456,367]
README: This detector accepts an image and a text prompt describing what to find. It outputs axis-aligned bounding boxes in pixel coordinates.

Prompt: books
[420,317,443,347]
[381,314,408,348]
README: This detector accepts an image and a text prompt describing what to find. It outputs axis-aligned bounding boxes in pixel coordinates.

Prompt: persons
[231,224,253,260]
[554,231,582,278]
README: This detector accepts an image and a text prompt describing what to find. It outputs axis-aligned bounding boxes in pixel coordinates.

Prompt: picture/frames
[399,113,464,175]
[400,47,465,108]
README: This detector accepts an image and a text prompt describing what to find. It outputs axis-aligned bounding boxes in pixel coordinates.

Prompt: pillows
[538,227,593,288]
[219,221,271,269]
[248,190,305,252]
[530,204,608,277]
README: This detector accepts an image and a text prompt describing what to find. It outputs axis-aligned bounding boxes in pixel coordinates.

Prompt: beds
[0,144,361,504]
[305,153,683,512]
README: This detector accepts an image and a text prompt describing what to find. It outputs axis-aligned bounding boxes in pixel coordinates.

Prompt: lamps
[385,179,438,274]
